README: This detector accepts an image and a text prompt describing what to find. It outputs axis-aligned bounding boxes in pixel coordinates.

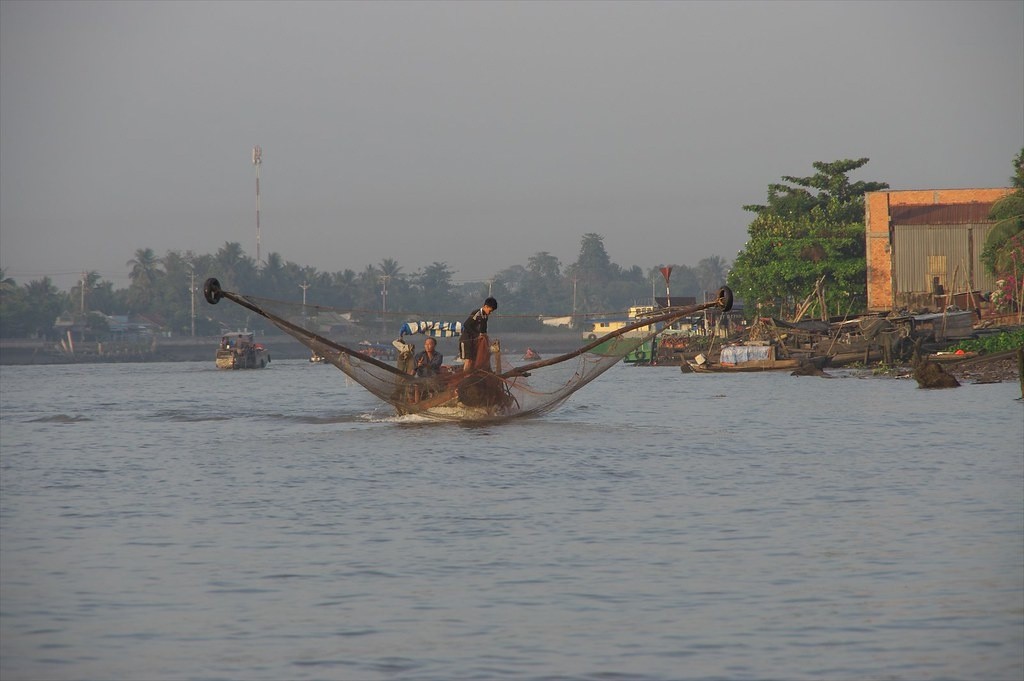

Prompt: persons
[221,333,244,357]
[413,337,443,379]
[459,297,497,373]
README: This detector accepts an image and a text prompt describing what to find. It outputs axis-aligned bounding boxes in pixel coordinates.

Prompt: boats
[213,331,272,369]
[621,285,1023,374]
[308,341,397,365]
[202,275,741,429]
